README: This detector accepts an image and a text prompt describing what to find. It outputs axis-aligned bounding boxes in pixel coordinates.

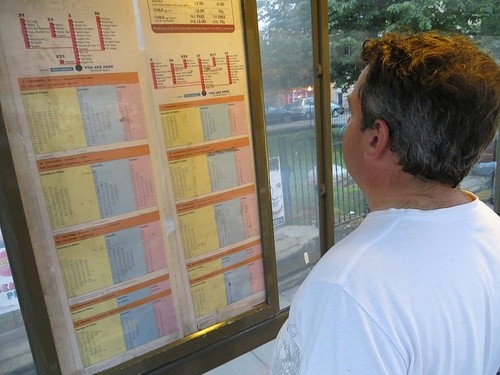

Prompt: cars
[264,103,305,124]
[281,97,344,121]
[459,162,496,210]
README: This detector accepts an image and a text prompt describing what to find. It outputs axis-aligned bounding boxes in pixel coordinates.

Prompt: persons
[273,32,500,375]
